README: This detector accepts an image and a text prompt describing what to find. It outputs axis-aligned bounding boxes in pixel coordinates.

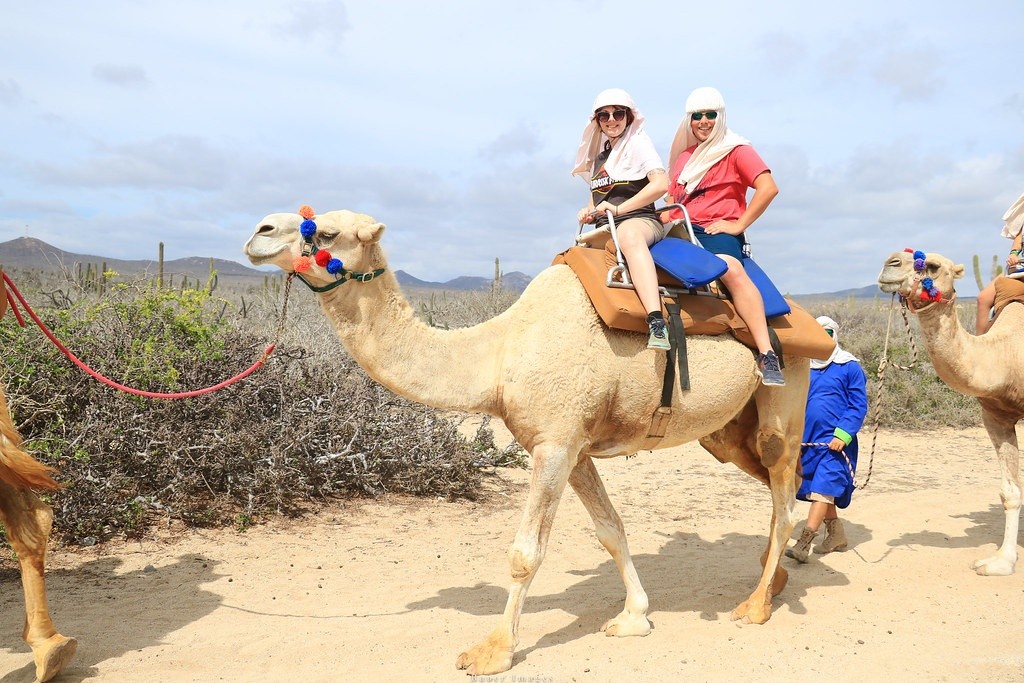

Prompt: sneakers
[647,317,671,351]
[756,351,786,387]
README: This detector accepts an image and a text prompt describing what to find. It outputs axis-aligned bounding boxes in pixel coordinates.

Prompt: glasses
[691,112,717,120]
[596,108,627,121]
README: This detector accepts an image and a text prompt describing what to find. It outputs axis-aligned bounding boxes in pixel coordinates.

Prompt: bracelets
[616,205,619,216]
[1010,249,1020,255]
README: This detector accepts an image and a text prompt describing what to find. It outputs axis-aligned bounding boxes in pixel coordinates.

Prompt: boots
[785,527,819,563]
[810,519,848,553]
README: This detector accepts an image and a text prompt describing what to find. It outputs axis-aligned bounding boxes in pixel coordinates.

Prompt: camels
[243,209,814,678]
[0,374,79,683]
[876,249,1022,575]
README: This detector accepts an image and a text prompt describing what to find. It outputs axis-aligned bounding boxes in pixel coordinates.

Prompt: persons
[659,85,787,388]
[783,314,868,564]
[577,87,672,350]
[974,192,1024,336]
